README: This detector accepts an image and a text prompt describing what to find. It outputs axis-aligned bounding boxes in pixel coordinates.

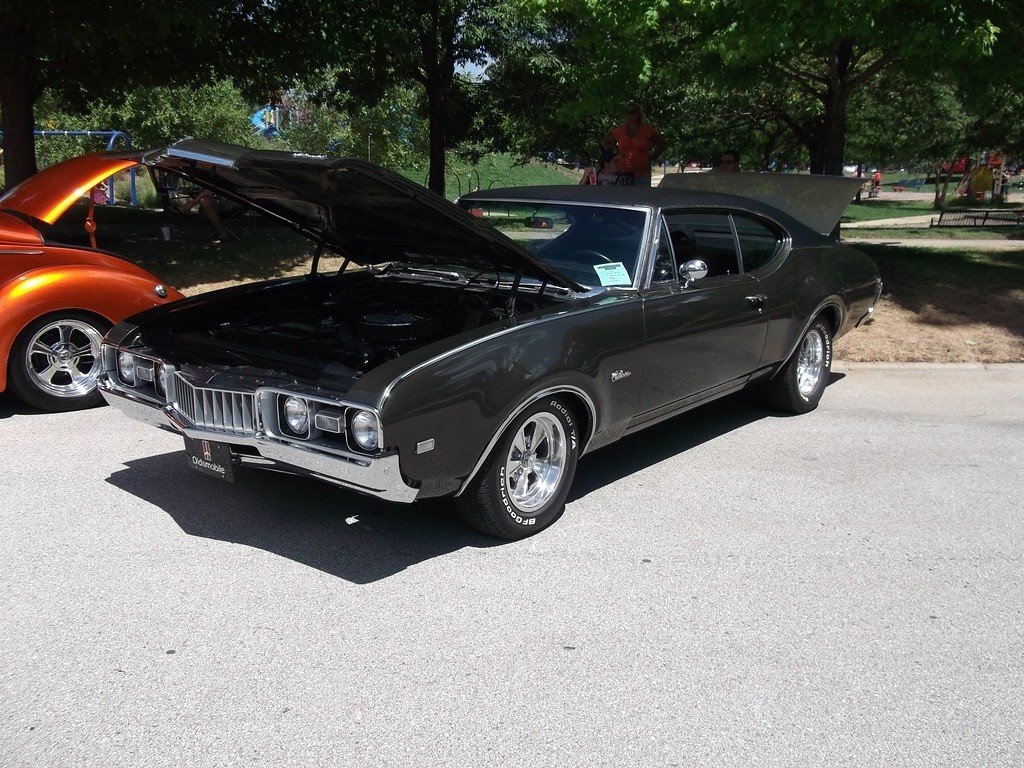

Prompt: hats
[597,147,625,169]
[670,224,697,244]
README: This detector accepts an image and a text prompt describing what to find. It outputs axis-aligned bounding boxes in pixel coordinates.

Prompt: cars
[939,156,968,174]
[0,152,190,414]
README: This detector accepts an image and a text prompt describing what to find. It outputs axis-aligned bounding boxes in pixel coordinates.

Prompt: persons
[716,150,740,173]
[578,102,668,189]
[167,189,242,243]
[93,181,109,204]
[853,167,1011,200]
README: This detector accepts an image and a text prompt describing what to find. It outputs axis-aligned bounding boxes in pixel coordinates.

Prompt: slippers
[167,204,191,216]
[208,237,233,245]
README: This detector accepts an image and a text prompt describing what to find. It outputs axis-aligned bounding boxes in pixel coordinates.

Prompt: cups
[162,227,170,241]
[587,167,597,185]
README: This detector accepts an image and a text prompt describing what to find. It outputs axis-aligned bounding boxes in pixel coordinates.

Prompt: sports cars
[93,137,884,541]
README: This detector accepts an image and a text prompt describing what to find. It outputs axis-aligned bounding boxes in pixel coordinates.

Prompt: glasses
[719,159,737,165]
[625,109,636,116]
